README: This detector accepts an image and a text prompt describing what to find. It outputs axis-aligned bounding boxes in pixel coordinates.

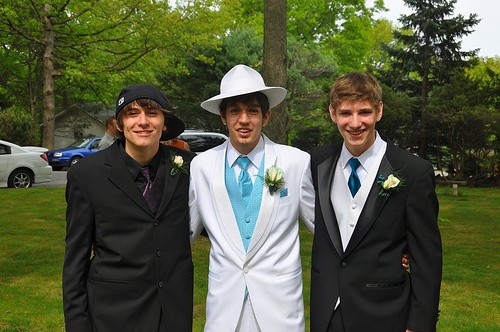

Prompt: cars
[46,137,103,171]
[0,140,54,188]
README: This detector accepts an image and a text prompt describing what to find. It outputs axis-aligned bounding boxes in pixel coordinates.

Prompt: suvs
[177,130,229,154]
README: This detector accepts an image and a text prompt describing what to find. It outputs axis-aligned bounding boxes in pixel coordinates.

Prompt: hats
[200,64,287,116]
[116,85,185,142]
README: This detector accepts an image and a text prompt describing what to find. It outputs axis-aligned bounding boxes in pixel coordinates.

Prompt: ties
[347,158,362,197]
[238,156,253,207]
[141,165,163,216]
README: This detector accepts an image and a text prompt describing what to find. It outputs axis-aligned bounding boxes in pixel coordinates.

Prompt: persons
[99,117,118,150]
[62,84,198,332]
[308,72,443,332]
[163,138,190,151]
[188,63,410,332]
